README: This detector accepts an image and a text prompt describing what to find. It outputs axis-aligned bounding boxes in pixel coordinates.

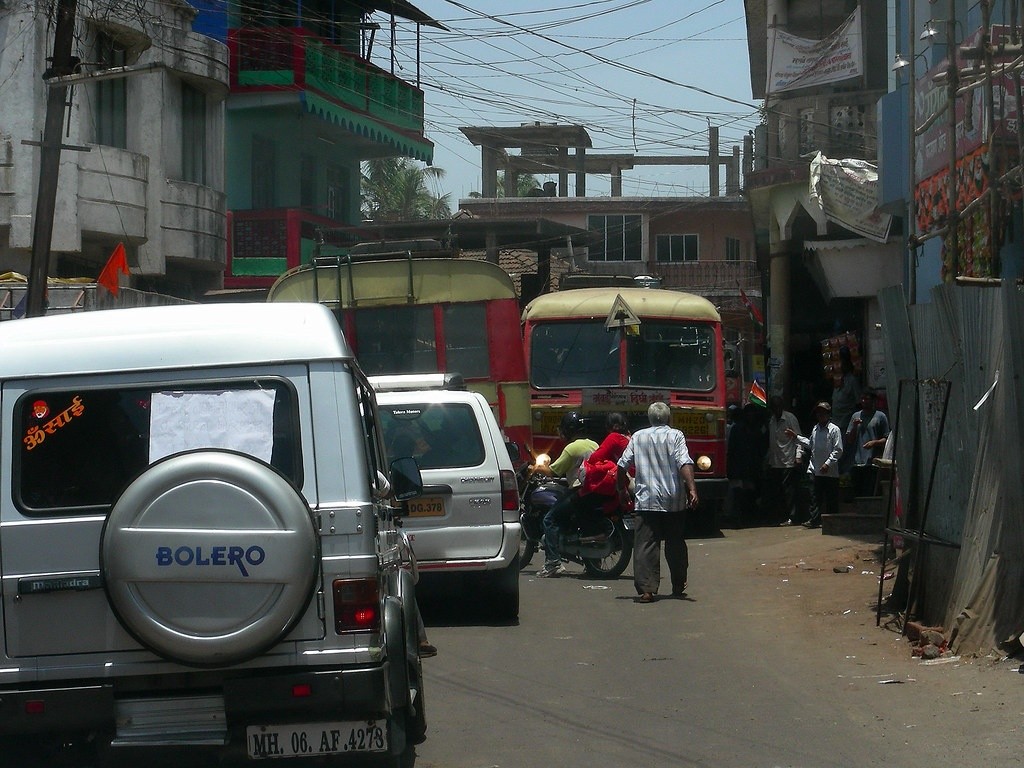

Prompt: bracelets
[617,488,624,491]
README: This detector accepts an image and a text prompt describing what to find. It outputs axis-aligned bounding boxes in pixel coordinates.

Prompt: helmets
[556,412,586,436]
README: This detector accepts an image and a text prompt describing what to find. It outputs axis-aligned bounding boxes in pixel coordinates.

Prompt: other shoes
[779,520,794,526]
[418,640,437,658]
[578,528,606,542]
[672,593,687,599]
[801,520,816,527]
[638,592,654,603]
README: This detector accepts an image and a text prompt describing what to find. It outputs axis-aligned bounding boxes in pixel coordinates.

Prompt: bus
[519,285,737,531]
[266,238,534,477]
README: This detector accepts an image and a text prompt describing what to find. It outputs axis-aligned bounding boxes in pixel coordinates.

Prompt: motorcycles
[518,451,638,581]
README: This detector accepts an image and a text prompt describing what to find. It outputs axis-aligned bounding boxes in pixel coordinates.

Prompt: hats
[816,401,832,412]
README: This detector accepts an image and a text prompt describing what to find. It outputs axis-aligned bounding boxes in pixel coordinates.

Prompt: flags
[740,289,765,342]
[748,380,766,408]
[100,245,130,299]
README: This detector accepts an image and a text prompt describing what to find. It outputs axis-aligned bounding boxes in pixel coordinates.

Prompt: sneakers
[536,558,567,578]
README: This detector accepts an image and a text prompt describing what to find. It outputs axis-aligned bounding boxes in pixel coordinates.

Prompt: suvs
[350,371,524,626]
[0,302,428,768]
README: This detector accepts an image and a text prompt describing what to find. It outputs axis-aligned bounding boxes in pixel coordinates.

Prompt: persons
[531,410,600,578]
[768,395,810,526]
[785,401,844,528]
[578,412,636,539]
[616,401,699,603]
[726,403,768,477]
[845,389,890,496]
